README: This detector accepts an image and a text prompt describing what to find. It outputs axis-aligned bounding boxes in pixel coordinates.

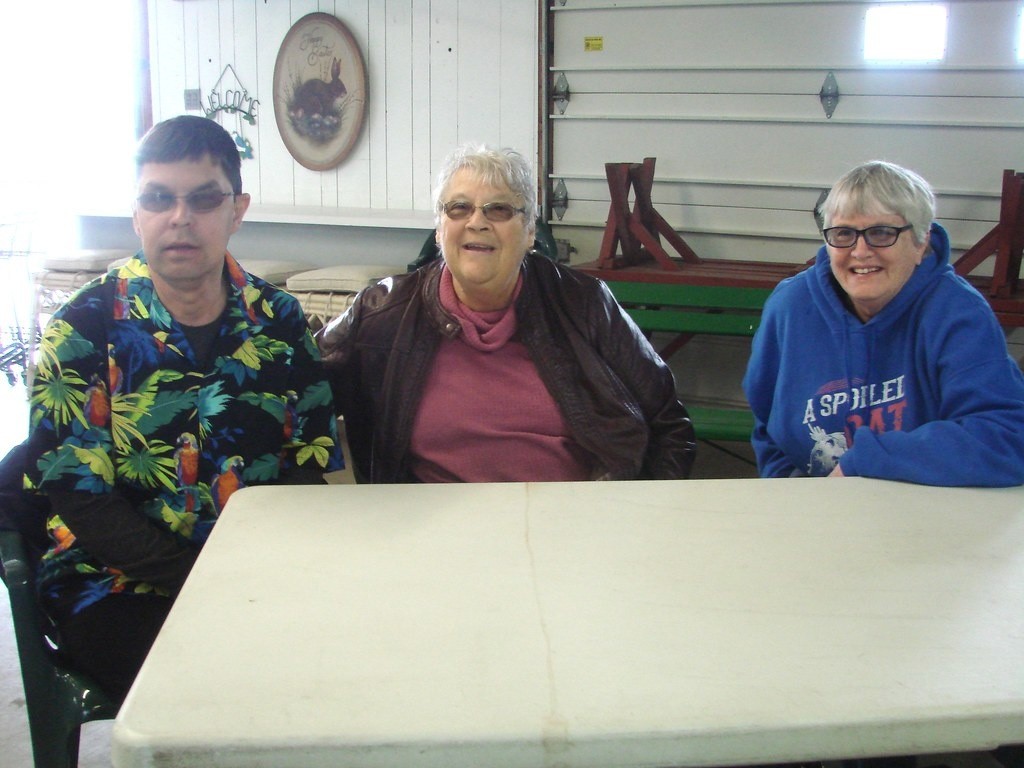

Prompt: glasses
[134,188,233,213]
[819,222,916,249]
[440,197,526,223]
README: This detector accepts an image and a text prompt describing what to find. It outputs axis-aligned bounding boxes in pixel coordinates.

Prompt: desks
[108,472,1022,767]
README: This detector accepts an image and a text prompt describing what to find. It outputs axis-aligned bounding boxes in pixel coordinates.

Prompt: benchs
[587,279,786,478]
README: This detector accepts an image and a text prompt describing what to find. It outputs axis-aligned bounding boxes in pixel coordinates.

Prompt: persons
[750,161,1023,496]
[313,145,697,487]
[1,115,345,765]
